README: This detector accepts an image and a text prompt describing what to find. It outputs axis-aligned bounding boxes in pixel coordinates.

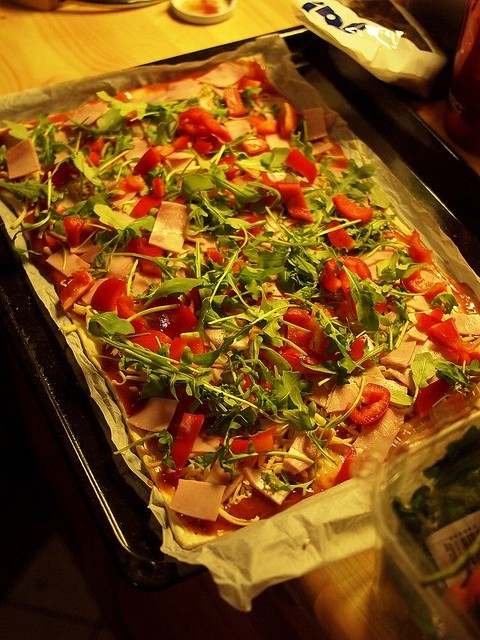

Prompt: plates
[166,0,236,27]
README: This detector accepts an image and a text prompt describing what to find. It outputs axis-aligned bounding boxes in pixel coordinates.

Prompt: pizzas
[0,52,480,549]
